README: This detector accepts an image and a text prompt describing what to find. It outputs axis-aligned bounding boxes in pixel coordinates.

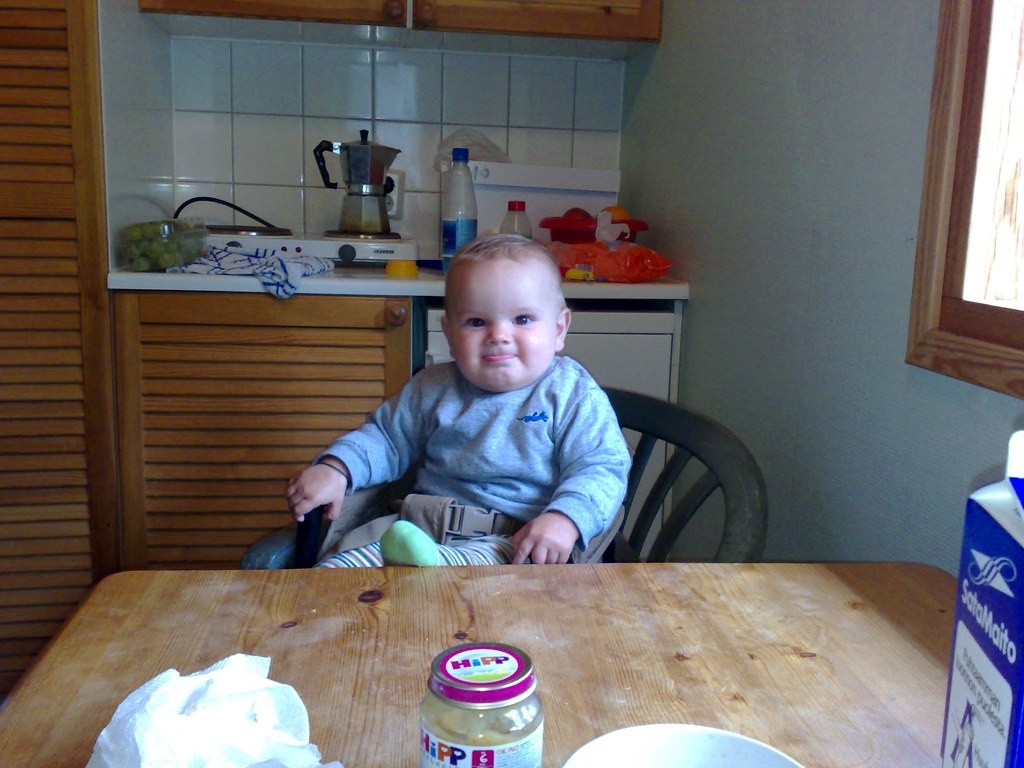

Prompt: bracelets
[316,462,348,479]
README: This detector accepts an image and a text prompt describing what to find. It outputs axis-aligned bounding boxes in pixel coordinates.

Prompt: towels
[165,242,335,301]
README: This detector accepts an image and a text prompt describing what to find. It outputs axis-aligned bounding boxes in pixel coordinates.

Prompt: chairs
[248,386,768,568]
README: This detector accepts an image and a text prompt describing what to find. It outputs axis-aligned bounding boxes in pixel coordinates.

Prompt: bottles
[499,201,532,240]
[441,148,477,276]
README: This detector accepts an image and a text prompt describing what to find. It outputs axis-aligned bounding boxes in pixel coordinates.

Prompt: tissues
[542,211,673,284]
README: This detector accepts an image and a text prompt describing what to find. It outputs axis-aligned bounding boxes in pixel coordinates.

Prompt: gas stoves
[181,222,418,269]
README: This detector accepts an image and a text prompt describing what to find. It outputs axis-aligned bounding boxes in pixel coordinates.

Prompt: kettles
[313,129,401,195]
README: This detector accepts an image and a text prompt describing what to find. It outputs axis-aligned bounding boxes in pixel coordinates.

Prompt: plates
[562,724,806,768]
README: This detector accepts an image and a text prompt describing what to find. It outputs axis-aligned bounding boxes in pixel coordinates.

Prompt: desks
[0,561,962,768]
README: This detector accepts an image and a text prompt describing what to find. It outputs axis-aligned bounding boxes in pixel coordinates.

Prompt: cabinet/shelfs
[0,0,693,696]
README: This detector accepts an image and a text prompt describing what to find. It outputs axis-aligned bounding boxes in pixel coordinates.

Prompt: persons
[284,234,631,566]
